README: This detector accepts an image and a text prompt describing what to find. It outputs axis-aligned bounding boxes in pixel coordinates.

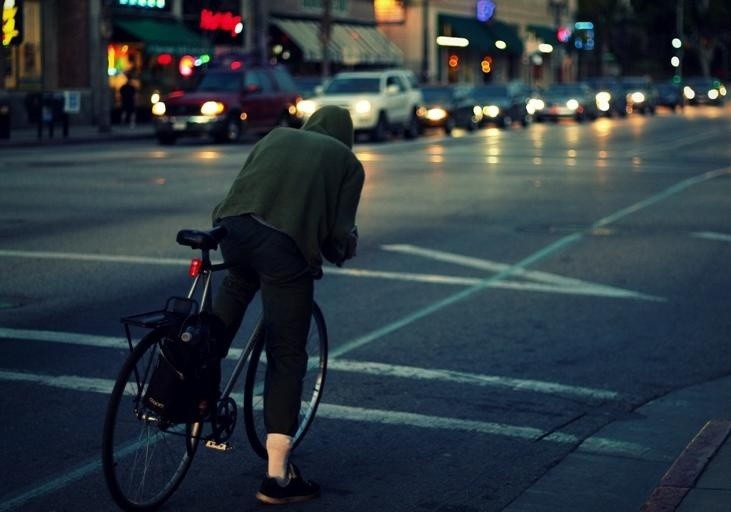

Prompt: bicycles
[100,227,330,511]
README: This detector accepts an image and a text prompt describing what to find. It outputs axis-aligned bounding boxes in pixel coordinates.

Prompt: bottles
[181,324,201,343]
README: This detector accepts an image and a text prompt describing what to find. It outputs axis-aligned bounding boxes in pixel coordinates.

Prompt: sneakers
[252,459,327,507]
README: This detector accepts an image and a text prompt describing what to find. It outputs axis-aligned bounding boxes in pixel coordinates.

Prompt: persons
[120,77,137,125]
[137,106,365,505]
